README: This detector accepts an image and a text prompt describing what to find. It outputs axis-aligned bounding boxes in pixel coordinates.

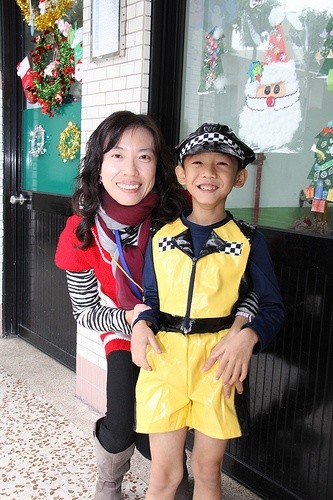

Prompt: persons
[130,124,286,500]
[54,111,259,500]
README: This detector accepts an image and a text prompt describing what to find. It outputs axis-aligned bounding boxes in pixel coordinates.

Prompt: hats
[174,120,255,170]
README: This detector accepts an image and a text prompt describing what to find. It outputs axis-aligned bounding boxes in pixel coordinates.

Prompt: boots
[92,416,135,500]
[173,452,190,500]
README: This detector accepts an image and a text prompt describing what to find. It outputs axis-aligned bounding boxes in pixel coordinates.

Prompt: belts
[157,311,235,335]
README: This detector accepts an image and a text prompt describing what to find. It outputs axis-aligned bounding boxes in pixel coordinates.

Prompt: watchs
[235,311,255,323]
